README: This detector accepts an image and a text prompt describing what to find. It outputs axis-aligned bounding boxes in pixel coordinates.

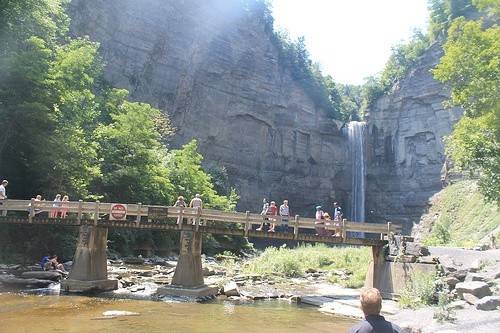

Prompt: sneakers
[256,228,261,230]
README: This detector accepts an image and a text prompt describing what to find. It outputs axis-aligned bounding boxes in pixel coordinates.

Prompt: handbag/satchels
[321,216,325,224]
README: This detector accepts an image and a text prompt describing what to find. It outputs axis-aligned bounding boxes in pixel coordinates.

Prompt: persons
[346,288,407,333]
[51,255,66,271]
[189,193,203,225]
[173,196,187,224]
[53,193,61,218]
[40,254,52,270]
[315,201,343,237]
[28,195,43,217]
[60,195,69,219]
[255,197,290,233]
[0,180,8,214]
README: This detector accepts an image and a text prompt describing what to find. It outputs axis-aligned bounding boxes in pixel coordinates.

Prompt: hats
[324,213,329,216]
[316,206,321,209]
